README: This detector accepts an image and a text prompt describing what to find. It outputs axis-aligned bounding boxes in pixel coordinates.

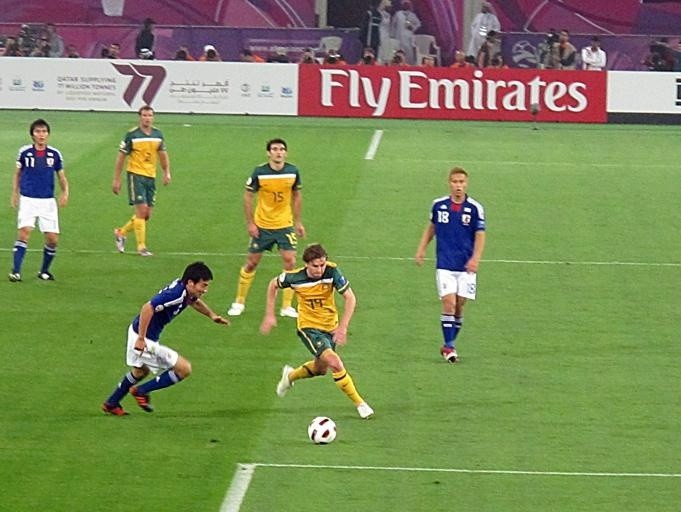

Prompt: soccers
[307,415,338,445]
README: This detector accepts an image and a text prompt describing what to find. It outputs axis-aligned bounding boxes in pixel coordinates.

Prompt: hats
[138,48,153,57]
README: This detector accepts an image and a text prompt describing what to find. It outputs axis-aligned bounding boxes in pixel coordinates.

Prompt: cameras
[100,47,109,57]
[43,44,51,57]
[207,49,217,58]
[363,55,373,65]
[304,55,312,64]
[392,54,402,65]
[546,32,560,47]
[328,53,338,65]
[176,50,187,59]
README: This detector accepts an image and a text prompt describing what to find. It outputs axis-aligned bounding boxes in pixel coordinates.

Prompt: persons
[466,2,501,59]
[171,44,196,60]
[268,46,293,63]
[299,46,319,63]
[0,21,81,58]
[135,18,157,60]
[100,262,230,413]
[356,46,380,66]
[449,48,476,68]
[323,48,346,65]
[539,29,577,70]
[112,106,172,257]
[239,50,265,62]
[416,167,487,362]
[390,48,410,66]
[419,56,434,67]
[100,42,121,59]
[259,244,375,419]
[476,30,498,68]
[581,36,607,72]
[639,35,674,72]
[7,119,70,282]
[199,44,221,61]
[227,139,307,318]
[358,0,392,66]
[388,0,421,65]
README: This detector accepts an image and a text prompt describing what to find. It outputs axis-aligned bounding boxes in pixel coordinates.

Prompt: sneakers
[439,345,449,357]
[444,348,458,364]
[129,386,155,413]
[226,303,246,316]
[279,307,300,319]
[7,271,23,282]
[358,400,375,420]
[113,228,128,254]
[276,365,294,399]
[36,271,55,281]
[101,400,130,418]
[137,247,152,257]
